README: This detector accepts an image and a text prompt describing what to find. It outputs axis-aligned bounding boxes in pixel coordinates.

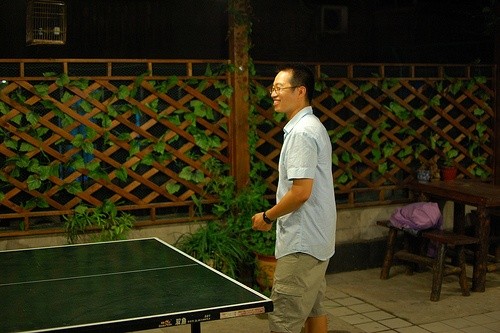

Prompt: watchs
[263,211,274,224]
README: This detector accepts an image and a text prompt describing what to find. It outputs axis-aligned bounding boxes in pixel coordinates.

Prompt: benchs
[376,220,479,302]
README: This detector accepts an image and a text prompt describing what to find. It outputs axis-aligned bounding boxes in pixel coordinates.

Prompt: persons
[250,67,337,333]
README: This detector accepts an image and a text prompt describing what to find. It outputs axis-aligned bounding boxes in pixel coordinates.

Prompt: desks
[0,237,274,333]
[400,180,500,292]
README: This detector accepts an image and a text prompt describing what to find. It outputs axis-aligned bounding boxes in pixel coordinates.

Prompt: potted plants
[175,179,277,291]
[442,158,457,181]
[415,160,432,182]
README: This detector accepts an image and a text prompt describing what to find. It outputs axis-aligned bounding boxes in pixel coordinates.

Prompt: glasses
[268,85,297,94]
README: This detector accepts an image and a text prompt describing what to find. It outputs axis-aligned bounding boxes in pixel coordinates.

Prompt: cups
[416,170,430,184]
